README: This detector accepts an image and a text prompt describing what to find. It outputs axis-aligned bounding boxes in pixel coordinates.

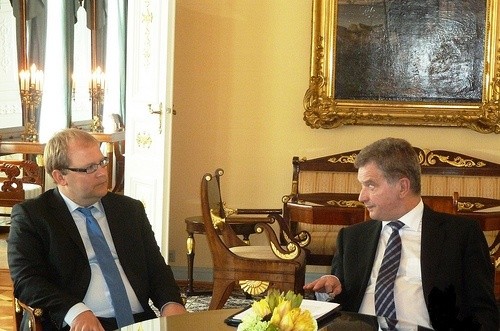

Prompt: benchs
[281,147,500,267]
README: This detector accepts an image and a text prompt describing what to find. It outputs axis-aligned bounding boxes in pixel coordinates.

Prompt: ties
[76,206,135,328]
[374,220,405,318]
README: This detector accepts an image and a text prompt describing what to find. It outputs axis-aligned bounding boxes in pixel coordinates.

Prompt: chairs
[200,167,311,311]
[0,160,45,227]
[12,285,44,331]
[489,255,499,300]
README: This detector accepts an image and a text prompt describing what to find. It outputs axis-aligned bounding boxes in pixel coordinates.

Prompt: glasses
[63,156,110,175]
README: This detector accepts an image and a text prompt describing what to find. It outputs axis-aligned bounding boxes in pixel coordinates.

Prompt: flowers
[236,289,319,331]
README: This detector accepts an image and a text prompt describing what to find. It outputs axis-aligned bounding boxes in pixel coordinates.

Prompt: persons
[7,128,189,331]
[302,137,497,331]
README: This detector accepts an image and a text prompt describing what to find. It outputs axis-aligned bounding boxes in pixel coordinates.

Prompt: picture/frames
[302,0,500,133]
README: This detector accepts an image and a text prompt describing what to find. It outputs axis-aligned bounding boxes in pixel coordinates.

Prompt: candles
[89,67,107,90]
[19,64,44,94]
[71,72,76,89]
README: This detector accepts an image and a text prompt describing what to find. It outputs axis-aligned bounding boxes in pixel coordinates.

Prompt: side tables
[184,214,258,297]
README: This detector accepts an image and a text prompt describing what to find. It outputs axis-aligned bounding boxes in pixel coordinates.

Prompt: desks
[113,309,433,331]
[281,191,500,268]
[86,130,124,192]
[0,138,49,166]
